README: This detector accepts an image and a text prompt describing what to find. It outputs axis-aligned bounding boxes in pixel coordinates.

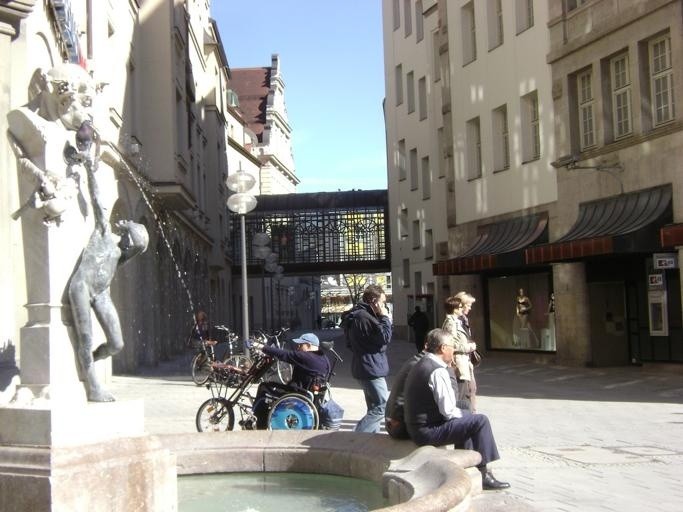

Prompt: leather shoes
[482,471,510,490]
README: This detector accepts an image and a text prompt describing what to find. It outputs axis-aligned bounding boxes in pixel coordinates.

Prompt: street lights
[224,159,259,367]
[264,251,285,333]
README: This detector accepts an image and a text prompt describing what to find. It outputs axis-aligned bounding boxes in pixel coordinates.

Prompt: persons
[341,284,393,433]
[248,332,331,430]
[66,152,149,402]
[384,291,510,489]
[516,287,540,348]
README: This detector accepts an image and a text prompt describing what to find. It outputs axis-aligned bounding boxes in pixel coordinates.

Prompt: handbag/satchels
[320,400,344,429]
[472,349,483,367]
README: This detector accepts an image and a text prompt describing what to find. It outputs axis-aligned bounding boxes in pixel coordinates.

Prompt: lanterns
[253,232,300,288]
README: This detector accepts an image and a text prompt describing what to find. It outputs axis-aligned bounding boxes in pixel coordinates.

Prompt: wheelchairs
[195,339,344,432]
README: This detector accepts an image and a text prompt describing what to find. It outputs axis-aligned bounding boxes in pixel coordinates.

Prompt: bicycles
[189,322,293,386]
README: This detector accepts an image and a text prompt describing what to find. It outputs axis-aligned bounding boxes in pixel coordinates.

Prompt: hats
[292,333,320,347]
